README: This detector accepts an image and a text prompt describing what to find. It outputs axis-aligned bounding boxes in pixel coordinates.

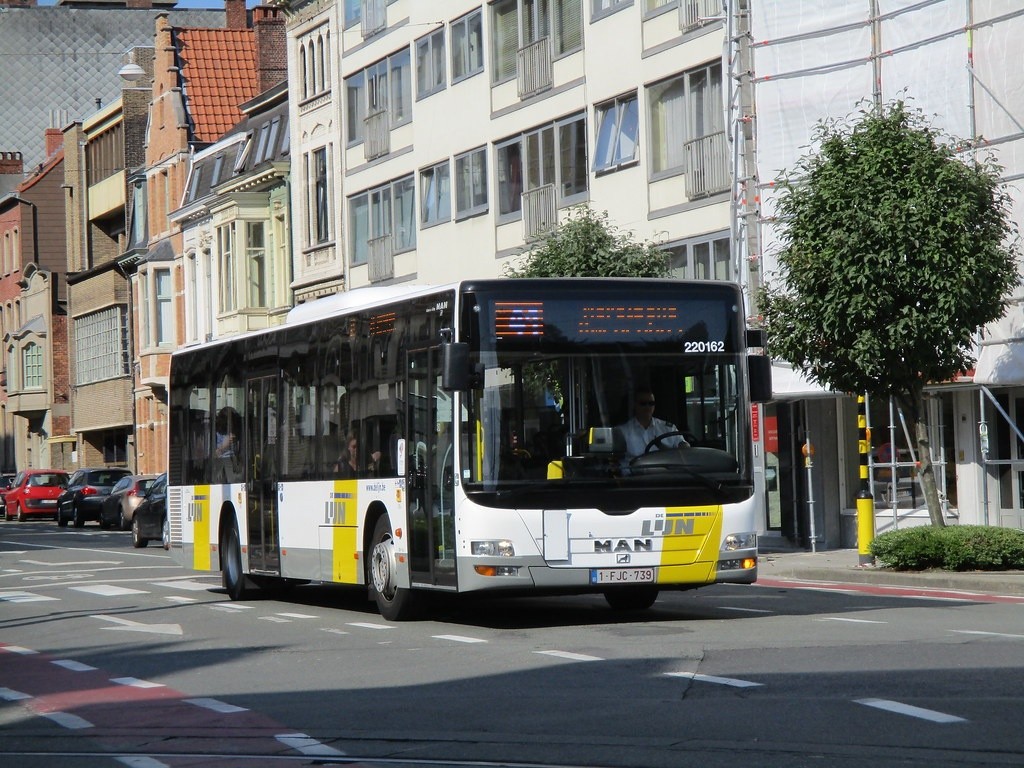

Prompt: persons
[216,407,242,457]
[333,428,375,473]
[615,390,690,475]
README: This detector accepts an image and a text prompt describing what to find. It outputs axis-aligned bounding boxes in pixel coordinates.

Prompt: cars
[131,472,169,550]
[98,474,161,530]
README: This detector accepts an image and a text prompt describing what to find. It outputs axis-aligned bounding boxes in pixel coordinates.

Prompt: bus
[167,278,773,621]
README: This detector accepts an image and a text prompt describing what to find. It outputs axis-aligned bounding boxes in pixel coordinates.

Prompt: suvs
[57,466,133,528]
[3,469,70,522]
[0,471,18,514]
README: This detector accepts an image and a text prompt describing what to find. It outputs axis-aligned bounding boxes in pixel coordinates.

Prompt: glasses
[199,416,210,421]
[635,400,656,407]
